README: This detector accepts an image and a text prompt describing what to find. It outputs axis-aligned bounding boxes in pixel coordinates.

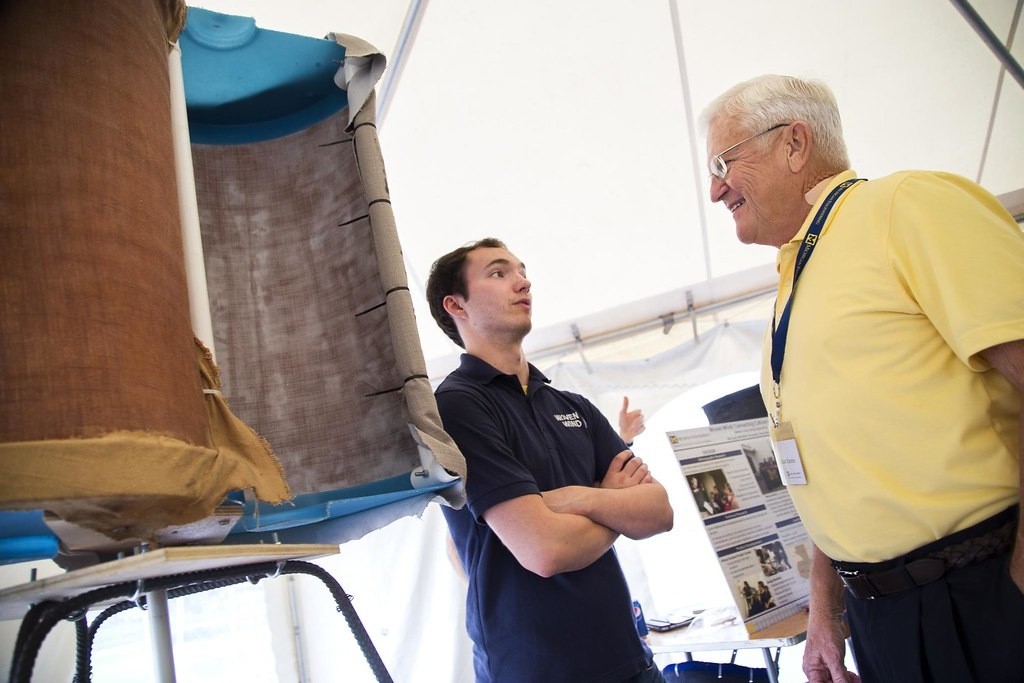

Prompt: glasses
[709,123,791,177]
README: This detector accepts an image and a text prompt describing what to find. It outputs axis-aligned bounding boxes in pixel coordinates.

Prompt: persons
[426,238,675,683]
[699,74,1024,683]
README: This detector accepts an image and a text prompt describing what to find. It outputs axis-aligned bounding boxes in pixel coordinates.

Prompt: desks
[647,607,809,683]
[1,542,394,683]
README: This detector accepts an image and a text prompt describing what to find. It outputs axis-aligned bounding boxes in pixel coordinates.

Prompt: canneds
[632,600,648,638]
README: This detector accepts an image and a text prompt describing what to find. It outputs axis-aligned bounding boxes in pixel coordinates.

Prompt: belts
[837,510,1020,601]
